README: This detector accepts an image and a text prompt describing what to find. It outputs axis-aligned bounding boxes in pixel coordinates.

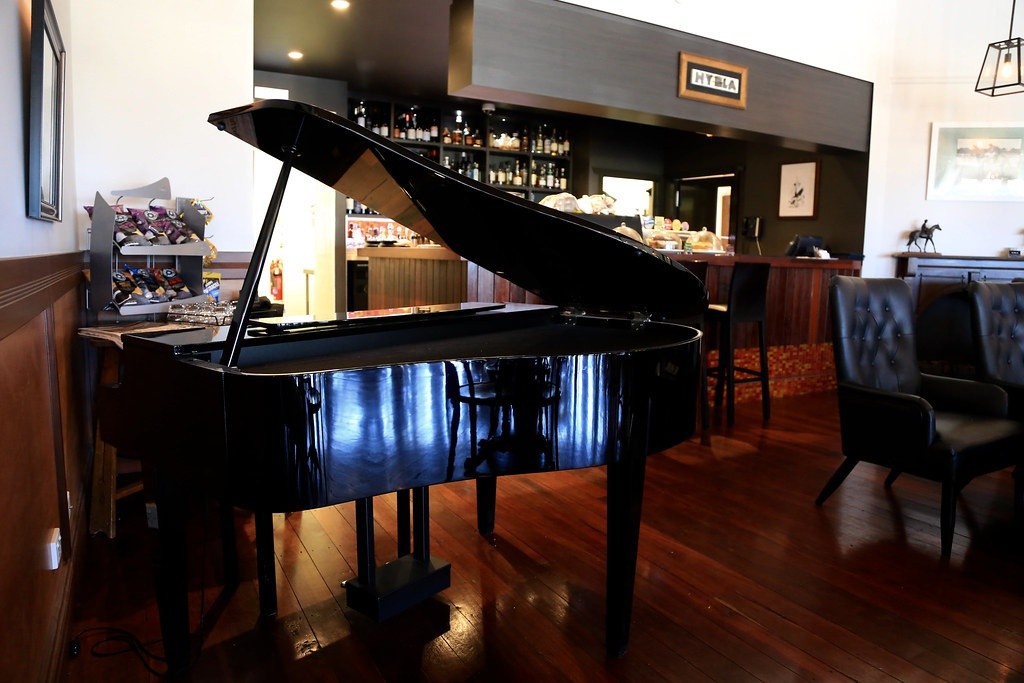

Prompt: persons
[922,220,930,238]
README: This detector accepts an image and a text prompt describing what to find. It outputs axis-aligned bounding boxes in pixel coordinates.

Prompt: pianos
[118,98,716,683]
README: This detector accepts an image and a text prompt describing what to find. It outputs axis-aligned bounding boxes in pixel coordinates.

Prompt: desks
[88,321,223,538]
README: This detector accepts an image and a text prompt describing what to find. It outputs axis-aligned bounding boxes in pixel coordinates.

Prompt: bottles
[346,98,569,248]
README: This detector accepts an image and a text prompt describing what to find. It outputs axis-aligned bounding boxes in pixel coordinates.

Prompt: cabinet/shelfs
[90,191,212,322]
[344,93,574,251]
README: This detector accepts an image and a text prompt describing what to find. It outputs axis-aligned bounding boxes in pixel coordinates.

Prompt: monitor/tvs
[785,234,823,257]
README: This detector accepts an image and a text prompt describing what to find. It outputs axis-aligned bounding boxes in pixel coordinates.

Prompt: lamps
[974,0,1024,97]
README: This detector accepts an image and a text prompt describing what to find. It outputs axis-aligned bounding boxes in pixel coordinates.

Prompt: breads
[692,241,713,249]
[649,240,665,249]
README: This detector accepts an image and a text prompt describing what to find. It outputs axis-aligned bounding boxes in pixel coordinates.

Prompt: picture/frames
[926,122,1024,203]
[776,161,820,220]
[23,0,67,225]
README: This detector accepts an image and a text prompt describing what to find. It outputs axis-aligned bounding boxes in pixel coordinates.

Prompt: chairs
[816,276,1024,560]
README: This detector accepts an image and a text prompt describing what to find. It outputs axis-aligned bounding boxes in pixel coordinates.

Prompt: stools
[666,261,713,447]
[705,261,772,429]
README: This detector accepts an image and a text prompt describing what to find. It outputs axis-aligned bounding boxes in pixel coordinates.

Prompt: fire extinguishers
[270,259,282,300]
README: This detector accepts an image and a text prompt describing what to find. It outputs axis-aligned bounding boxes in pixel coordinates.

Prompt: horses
[906,225,942,253]
